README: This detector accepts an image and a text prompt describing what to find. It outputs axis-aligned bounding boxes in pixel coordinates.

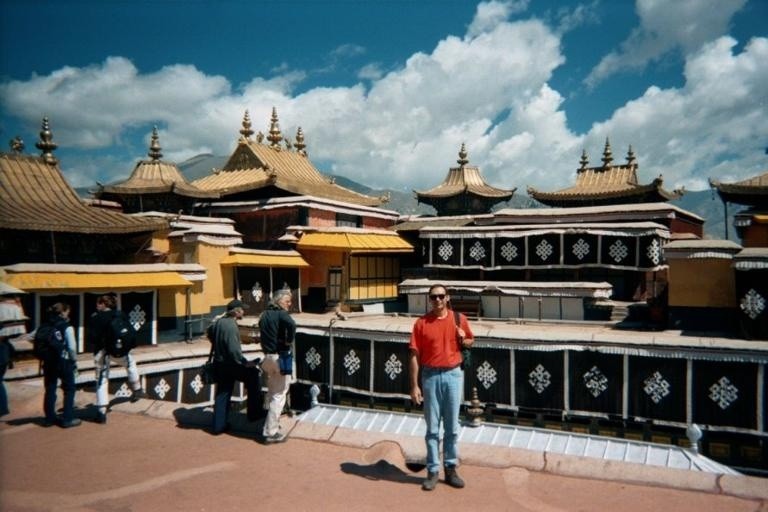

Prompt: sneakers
[260,433,284,443]
[45,414,83,428]
[88,411,107,423]
[129,387,144,403]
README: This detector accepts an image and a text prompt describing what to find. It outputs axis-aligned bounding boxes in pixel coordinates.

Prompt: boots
[421,468,439,491]
[443,464,465,487]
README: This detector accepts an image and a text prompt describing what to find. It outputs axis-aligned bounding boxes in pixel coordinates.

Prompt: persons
[406,284,474,491]
[24,302,81,428]
[0,319,15,418]
[85,295,146,423]
[205,299,267,435]
[257,288,296,445]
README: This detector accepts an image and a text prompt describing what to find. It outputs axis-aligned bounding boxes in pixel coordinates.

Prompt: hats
[226,299,252,310]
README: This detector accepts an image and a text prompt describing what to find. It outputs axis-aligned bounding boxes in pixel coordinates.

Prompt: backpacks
[98,312,138,358]
[33,318,70,365]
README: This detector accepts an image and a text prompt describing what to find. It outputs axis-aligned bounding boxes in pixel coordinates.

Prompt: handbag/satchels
[454,310,472,371]
[198,360,216,385]
[277,353,293,376]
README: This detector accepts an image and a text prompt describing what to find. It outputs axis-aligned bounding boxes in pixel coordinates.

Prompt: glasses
[428,293,445,301]
